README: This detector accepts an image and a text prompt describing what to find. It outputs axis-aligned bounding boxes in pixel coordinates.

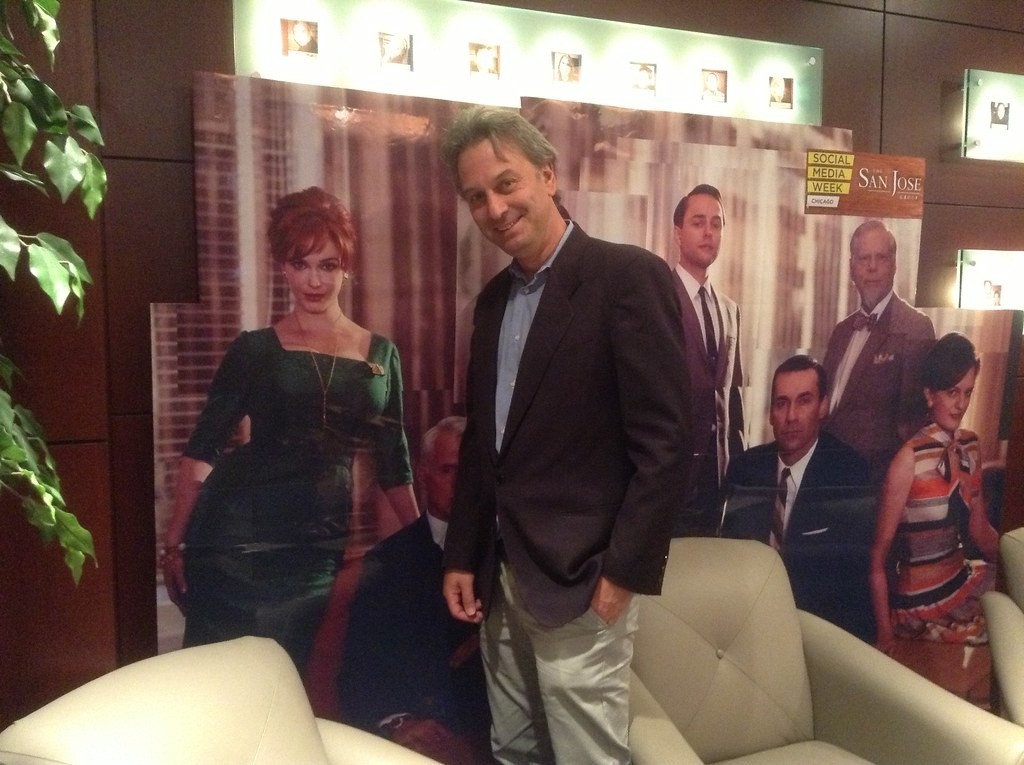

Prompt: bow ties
[852,312,879,332]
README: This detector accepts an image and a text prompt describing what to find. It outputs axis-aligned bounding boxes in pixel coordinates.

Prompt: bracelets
[158,543,186,566]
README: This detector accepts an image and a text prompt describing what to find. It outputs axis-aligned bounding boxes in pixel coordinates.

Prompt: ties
[698,286,718,367]
[769,467,791,550]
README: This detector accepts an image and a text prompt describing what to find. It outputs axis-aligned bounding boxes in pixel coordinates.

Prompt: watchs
[380,713,411,740]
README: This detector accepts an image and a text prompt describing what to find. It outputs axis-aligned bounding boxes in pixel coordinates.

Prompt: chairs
[0,636,451,765]
[627,526,1024,765]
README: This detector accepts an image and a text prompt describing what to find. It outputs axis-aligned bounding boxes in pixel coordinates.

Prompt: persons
[821,221,935,490]
[338,415,491,765]
[984,280,1000,305]
[161,188,419,693]
[292,21,790,104]
[443,109,687,765]
[671,184,746,538]
[869,332,1005,658]
[992,102,1008,124]
[722,355,877,643]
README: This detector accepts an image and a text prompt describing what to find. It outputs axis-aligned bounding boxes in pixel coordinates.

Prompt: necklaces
[292,306,343,423]
